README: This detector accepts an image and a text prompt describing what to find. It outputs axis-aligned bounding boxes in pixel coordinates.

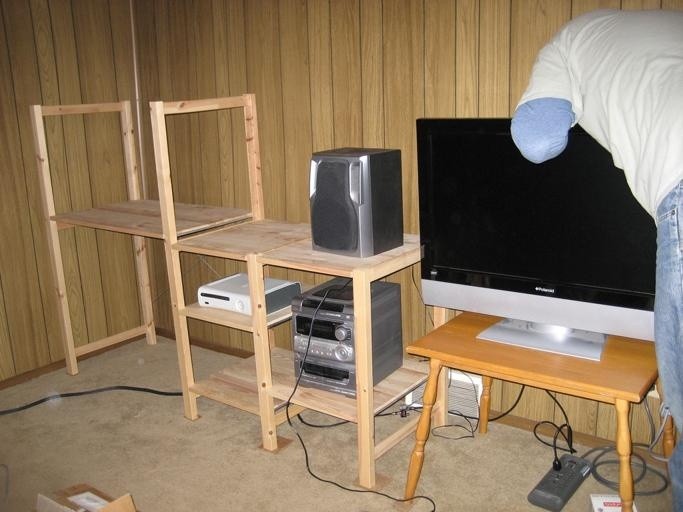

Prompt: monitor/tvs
[415,117,657,363]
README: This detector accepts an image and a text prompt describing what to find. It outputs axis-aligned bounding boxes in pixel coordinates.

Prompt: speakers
[308,146,404,259]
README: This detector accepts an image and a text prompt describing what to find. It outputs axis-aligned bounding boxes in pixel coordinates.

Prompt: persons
[511,10,683,512]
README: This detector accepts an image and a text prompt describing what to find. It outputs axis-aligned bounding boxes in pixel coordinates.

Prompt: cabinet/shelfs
[28,92,453,492]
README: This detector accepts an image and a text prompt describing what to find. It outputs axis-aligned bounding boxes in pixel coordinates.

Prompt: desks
[401,312,678,512]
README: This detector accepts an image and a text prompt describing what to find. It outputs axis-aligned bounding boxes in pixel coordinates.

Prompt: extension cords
[527,453,594,512]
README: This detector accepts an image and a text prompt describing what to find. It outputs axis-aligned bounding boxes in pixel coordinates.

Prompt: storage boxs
[34,483,138,512]
